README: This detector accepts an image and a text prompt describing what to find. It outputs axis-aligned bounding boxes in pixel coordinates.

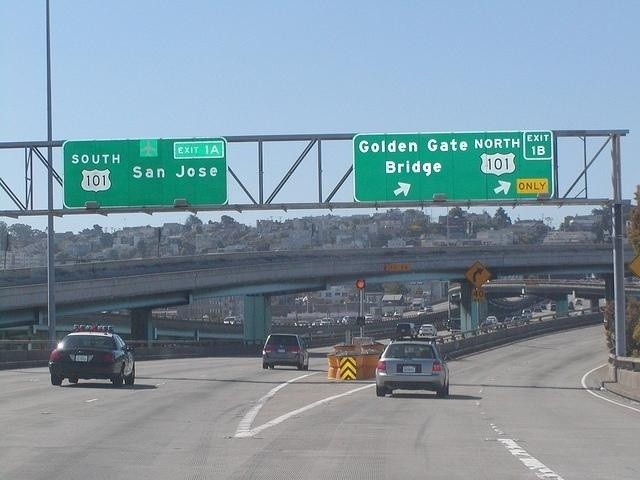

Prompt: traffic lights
[356,279,365,289]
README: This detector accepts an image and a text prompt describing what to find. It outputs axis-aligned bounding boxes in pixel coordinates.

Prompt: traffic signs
[353,130,556,203]
[62,140,227,211]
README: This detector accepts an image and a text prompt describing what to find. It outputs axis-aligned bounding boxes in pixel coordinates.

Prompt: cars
[418,304,432,316]
[534,304,556,312]
[397,323,438,338]
[569,298,581,310]
[262,334,309,371]
[374,337,450,397]
[48,322,136,386]
[485,308,532,325]
[296,317,349,327]
[366,311,401,319]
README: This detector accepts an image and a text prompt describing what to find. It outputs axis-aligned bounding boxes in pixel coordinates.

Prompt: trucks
[224,315,241,325]
